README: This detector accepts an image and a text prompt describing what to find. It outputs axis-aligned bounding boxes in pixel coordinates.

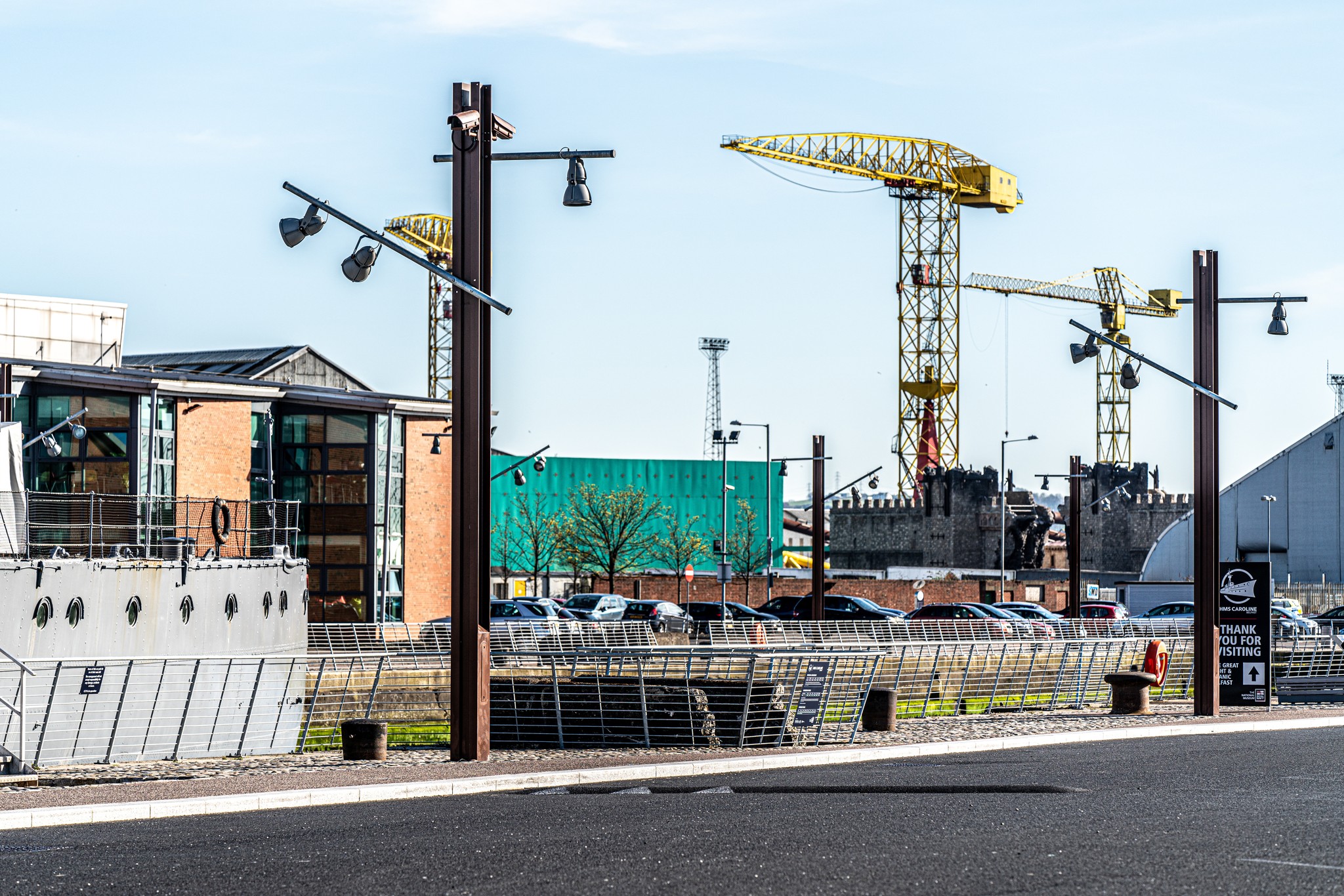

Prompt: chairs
[40,470,99,494]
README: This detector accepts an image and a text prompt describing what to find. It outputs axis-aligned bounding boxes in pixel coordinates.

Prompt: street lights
[1261,495,1277,711]
[1000,435,1039,602]
[1068,249,1309,717]
[1034,455,1133,622]
[277,84,616,762]
[771,435,883,651]
[1051,600,1196,633]
[712,430,741,622]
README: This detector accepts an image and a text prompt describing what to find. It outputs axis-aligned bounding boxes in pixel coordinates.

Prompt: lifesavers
[211,498,231,546]
[1156,653,1165,684]
[750,623,766,649]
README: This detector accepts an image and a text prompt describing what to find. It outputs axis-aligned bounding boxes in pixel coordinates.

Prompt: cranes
[381,213,453,405]
[963,264,1181,468]
[719,133,1025,505]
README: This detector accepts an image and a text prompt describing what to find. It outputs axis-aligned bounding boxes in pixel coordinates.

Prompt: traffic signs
[1219,561,1271,708]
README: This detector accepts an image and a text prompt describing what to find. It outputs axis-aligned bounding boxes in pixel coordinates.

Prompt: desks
[97,475,112,493]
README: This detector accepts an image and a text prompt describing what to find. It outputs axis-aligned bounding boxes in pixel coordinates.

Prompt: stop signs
[684,563,695,583]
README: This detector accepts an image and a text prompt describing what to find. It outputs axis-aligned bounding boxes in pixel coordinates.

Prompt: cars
[1271,598,1344,640]
[902,601,1070,639]
[621,598,697,636]
[418,592,631,652]
[752,593,908,642]
[678,602,778,633]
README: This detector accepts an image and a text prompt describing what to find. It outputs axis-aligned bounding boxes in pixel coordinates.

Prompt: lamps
[491,426,497,437]
[445,417,452,431]
[186,397,203,412]
